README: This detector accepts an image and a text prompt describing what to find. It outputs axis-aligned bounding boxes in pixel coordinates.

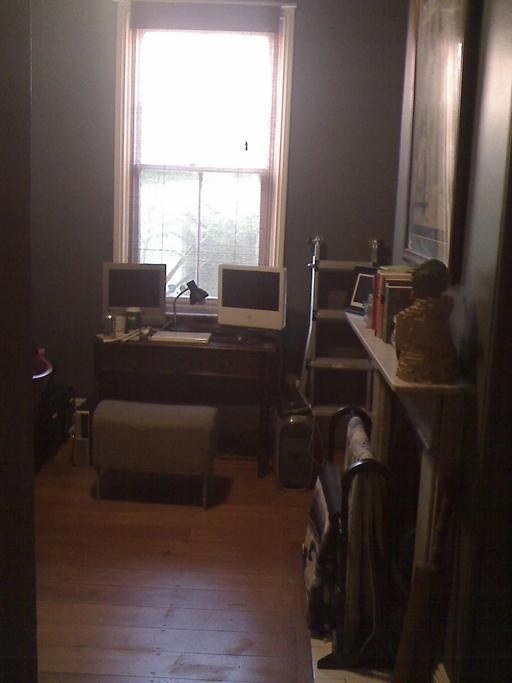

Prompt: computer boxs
[276,415,315,490]
[75,396,94,466]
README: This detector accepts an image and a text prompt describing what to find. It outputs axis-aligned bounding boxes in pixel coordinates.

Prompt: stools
[88,398,221,510]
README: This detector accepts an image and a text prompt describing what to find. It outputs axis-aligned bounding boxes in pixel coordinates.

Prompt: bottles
[140,324,152,341]
[395,299,450,381]
[103,310,116,337]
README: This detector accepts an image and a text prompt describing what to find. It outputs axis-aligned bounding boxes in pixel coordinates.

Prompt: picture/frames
[398,0,484,288]
[344,264,379,315]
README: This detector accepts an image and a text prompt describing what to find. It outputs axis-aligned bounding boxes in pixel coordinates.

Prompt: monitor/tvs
[101,263,166,326]
[217,264,287,332]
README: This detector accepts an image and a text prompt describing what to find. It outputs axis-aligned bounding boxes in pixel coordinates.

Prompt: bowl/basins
[33,354,53,395]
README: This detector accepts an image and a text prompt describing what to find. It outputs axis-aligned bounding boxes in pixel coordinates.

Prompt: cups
[125,307,141,333]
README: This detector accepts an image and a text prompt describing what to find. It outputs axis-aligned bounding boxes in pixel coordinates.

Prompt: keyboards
[150,331,212,343]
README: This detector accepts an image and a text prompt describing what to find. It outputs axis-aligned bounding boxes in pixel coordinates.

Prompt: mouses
[232,333,248,344]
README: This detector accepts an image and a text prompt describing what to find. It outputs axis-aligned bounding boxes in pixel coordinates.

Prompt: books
[369,265,415,344]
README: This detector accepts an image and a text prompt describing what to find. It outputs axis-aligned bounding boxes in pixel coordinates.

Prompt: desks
[91,310,282,480]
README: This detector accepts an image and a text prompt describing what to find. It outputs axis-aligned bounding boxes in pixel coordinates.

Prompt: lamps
[169,279,210,330]
